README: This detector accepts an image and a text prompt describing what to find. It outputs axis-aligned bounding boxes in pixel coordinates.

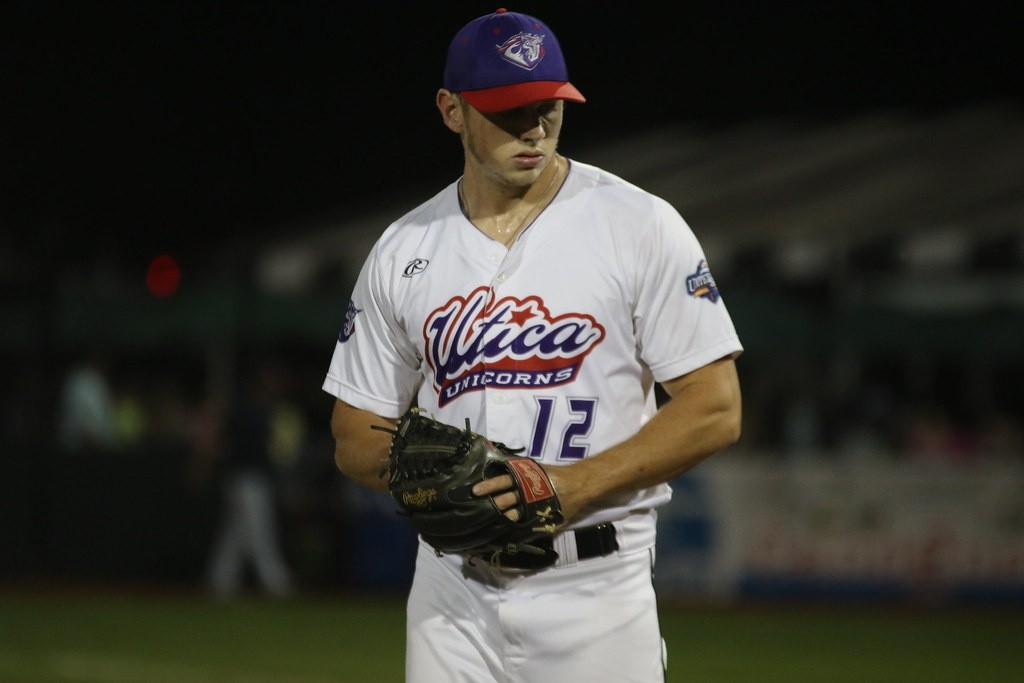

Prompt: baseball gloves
[378,399,581,598]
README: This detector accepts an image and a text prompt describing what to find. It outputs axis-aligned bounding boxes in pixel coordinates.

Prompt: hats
[443,8,586,113]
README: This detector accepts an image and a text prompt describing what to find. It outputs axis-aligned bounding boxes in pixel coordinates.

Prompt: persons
[205,362,975,604]
[322,9,745,683]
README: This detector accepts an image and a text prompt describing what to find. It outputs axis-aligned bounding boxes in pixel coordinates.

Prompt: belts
[471,521,620,570]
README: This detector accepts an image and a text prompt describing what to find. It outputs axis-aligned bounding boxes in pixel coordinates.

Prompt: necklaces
[461,155,560,243]
